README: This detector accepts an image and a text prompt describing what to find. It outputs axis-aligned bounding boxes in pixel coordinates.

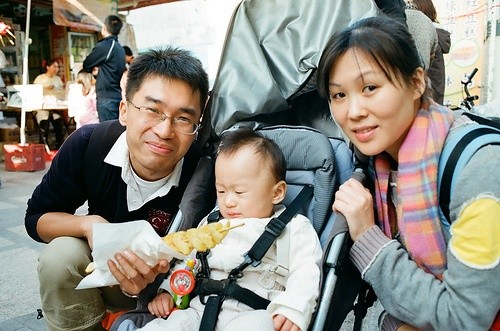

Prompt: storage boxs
[3,144,46,172]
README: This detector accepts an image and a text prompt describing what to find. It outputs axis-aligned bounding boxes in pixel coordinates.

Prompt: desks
[1,102,74,154]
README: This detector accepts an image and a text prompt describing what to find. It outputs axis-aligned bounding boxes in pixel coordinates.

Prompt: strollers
[111,0,451,331]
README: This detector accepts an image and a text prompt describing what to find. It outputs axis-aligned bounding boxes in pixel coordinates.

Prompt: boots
[54,117,65,150]
[39,119,49,144]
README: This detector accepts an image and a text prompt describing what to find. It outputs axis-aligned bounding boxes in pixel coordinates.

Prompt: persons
[374,0,450,105]
[84,15,127,121]
[24,45,210,330]
[31,60,67,149]
[72,69,100,130]
[122,46,136,64]
[136,128,322,331]
[316,16,500,331]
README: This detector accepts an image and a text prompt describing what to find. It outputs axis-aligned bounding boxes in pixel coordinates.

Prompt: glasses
[126,97,204,136]
